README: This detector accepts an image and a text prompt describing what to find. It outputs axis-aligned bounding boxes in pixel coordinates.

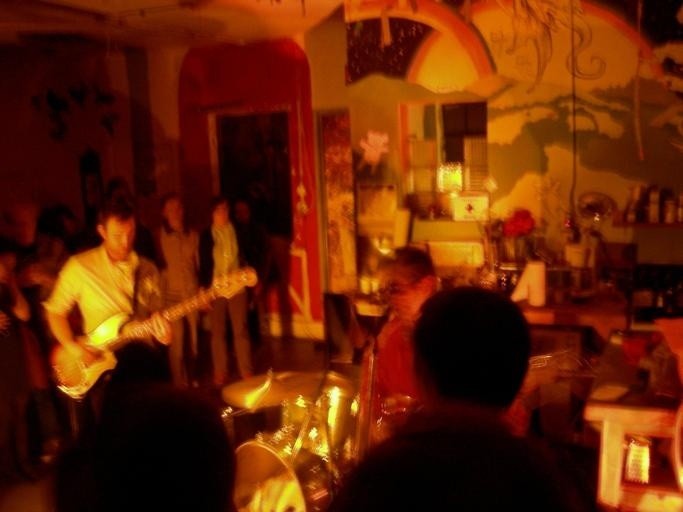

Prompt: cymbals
[220,373,330,410]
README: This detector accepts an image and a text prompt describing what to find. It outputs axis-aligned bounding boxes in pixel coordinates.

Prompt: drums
[233,430,339,512]
[281,378,356,453]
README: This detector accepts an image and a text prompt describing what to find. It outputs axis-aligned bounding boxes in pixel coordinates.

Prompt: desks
[585,335,683,508]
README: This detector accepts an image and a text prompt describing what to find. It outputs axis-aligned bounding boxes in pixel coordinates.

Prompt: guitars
[51,269,257,400]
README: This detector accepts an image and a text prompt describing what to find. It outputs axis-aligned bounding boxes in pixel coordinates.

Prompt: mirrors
[399,96,491,219]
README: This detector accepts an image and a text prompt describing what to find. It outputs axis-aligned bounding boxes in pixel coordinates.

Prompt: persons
[226,197,266,343]
[88,177,163,269]
[155,193,211,392]
[331,245,530,451]
[53,385,236,511]
[199,196,265,387]
[43,197,172,408]
[331,284,576,512]
[3,202,98,481]
